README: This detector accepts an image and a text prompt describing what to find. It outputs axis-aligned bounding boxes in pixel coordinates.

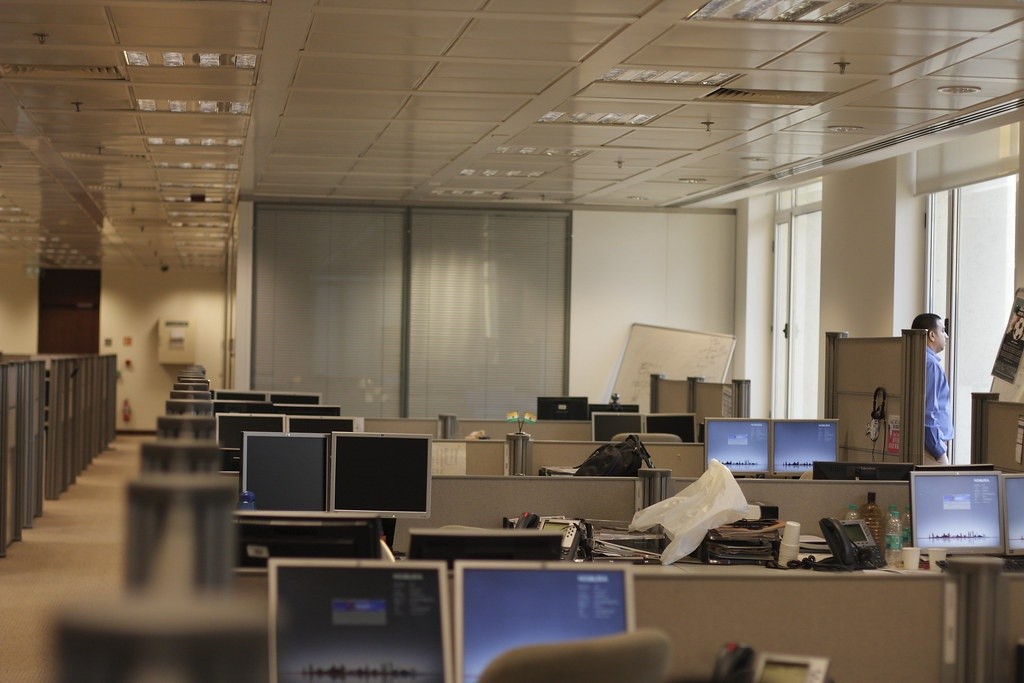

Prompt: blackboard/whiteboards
[608,322,737,414]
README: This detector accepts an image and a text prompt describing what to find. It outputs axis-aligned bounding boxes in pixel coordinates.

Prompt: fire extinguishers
[122,398,131,422]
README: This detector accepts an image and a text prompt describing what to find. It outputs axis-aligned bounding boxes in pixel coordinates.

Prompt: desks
[1,351,1024,683]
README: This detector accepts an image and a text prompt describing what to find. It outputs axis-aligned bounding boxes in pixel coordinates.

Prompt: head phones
[871,387,887,420]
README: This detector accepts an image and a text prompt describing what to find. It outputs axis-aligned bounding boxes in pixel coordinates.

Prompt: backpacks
[573,435,655,476]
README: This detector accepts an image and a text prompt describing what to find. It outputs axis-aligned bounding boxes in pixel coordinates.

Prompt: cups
[778,521,801,566]
[929,547,949,571]
[901,547,921,569]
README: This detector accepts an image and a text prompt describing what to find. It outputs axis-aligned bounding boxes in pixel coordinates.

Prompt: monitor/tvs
[703,415,1024,574]
[214,388,696,683]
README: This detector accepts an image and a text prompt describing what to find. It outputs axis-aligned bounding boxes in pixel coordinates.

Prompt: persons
[912,313,953,465]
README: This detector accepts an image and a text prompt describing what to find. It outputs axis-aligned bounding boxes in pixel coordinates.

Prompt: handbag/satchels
[628,459,760,566]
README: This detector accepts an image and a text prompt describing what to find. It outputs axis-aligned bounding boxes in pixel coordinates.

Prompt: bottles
[902,505,915,546]
[859,492,882,551]
[237,491,256,511]
[886,512,903,565]
[845,504,858,520]
[883,504,898,545]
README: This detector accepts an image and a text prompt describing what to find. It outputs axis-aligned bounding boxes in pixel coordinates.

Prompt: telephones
[514,512,583,561]
[819,517,887,571]
[709,643,831,682]
